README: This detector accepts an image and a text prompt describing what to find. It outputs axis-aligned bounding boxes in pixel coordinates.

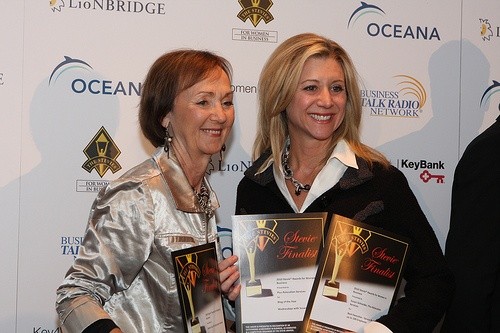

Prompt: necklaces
[282,144,311,196]
[190,183,213,218]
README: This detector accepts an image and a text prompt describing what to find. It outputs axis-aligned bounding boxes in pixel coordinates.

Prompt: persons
[54,48,236,333]
[444,103,500,333]
[217,33,447,333]
